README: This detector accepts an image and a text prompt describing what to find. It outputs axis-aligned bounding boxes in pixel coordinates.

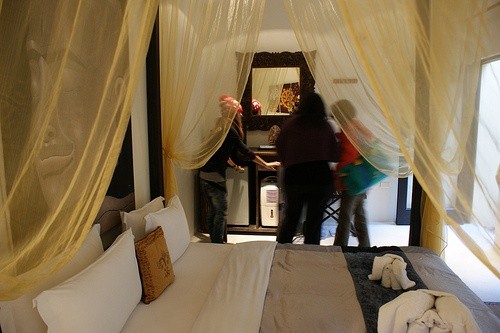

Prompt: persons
[251,98,262,116]
[329,99,373,248]
[275,93,343,245]
[200,96,277,244]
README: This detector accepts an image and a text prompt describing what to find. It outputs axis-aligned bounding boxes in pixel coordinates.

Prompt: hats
[220,95,242,114]
[252,98,261,111]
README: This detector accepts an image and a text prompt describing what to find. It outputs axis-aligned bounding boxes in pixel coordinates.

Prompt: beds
[92,193,500,333]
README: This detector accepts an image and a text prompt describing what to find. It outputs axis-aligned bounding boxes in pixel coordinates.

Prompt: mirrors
[234,49,317,132]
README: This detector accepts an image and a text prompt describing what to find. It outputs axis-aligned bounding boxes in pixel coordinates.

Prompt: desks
[318,194,366,235]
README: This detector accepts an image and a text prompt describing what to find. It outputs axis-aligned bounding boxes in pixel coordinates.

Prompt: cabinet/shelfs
[198,147,280,234]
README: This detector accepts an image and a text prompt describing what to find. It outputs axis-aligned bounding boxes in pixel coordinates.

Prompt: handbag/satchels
[337,142,393,196]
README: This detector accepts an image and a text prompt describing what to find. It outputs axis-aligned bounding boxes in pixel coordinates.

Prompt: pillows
[0,194,191,333]
